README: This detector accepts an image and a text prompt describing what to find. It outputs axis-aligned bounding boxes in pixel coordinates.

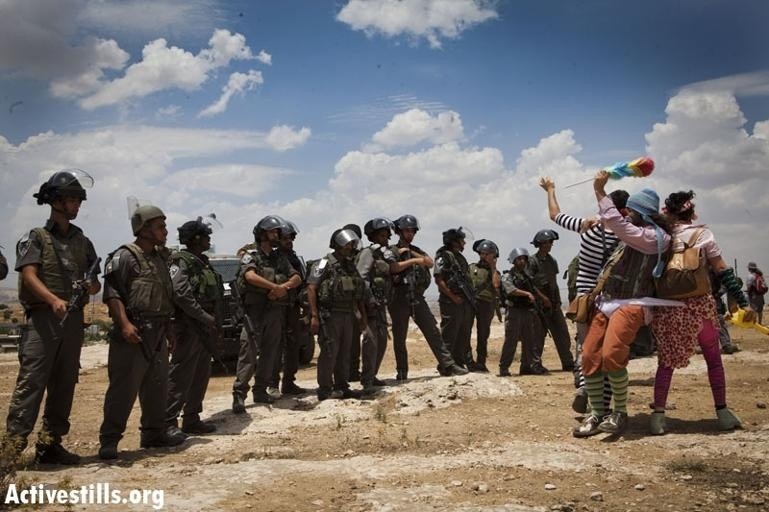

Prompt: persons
[97,205,184,459]
[307,229,377,402]
[536,172,769,437]
[495,246,545,377]
[468,238,501,371]
[352,216,391,394]
[433,226,485,375]
[228,215,303,414]
[0,167,102,465]
[526,228,575,372]
[0,250,10,281]
[343,224,363,382]
[162,220,227,441]
[387,214,470,380]
[265,219,308,398]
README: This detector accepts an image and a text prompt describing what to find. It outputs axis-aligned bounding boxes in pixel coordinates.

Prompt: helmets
[127,195,167,238]
[330,215,558,264]
[280,222,301,240]
[178,217,225,246]
[33,168,95,206]
[253,216,288,244]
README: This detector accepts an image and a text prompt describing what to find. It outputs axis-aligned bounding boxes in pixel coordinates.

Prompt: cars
[209,253,317,375]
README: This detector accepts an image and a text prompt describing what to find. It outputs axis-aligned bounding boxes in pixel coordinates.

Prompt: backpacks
[655,228,711,301]
[756,273,768,295]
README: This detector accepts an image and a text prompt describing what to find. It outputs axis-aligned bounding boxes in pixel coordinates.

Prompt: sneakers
[570,390,667,437]
[717,409,741,430]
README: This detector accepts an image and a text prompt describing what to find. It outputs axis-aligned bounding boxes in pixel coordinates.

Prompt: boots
[35,443,81,465]
[98,372,407,461]
[721,343,738,354]
[442,356,487,377]
[500,366,575,377]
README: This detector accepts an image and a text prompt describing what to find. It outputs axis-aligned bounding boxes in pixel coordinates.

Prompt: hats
[747,263,759,270]
[626,189,660,220]
